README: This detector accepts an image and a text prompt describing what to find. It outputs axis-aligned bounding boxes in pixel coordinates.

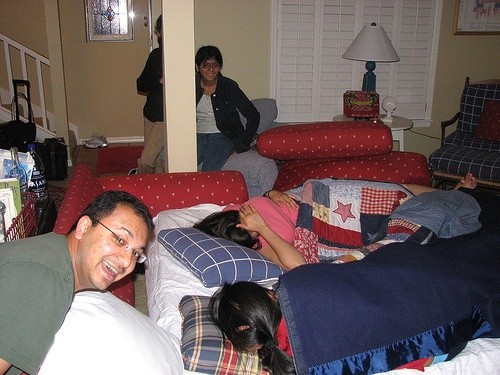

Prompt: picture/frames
[84,0,135,43]
[453,0,500,35]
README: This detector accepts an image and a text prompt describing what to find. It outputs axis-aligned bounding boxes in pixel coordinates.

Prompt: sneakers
[82,135,108,148]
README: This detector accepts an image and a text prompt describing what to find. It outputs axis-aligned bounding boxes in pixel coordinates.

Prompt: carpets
[97,146,145,173]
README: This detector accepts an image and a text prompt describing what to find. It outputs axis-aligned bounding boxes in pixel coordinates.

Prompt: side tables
[333,114,414,152]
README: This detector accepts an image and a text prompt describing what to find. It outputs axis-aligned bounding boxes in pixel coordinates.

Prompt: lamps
[342,22,400,91]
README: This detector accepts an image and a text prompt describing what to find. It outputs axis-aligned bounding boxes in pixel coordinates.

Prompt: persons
[127,15,166,175]
[0,190,154,375]
[195,45,260,172]
[191,171,500,375]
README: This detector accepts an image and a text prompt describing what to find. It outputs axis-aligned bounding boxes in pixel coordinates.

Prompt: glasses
[90,217,147,263]
[199,62,219,69]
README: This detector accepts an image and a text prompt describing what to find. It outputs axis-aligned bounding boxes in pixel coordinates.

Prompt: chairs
[427,76,500,192]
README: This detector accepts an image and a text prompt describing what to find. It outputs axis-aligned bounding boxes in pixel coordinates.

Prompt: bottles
[6,146,28,208]
[28,143,48,202]
[0,201,7,244]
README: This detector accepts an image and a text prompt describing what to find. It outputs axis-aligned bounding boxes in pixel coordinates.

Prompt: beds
[146,204,500,375]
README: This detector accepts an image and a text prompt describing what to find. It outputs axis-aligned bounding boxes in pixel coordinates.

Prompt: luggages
[41,137,69,180]
[0,80,36,152]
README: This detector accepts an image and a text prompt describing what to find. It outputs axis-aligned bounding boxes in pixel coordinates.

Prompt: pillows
[474,100,500,141]
[157,227,288,288]
[179,294,272,375]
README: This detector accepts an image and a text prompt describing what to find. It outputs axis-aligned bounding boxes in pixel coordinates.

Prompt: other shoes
[128,168,138,176]
